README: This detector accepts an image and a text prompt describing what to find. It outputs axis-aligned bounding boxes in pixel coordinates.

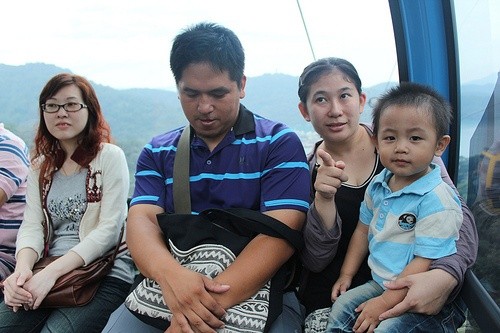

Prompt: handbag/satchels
[123,126,305,333]
[0,251,114,307]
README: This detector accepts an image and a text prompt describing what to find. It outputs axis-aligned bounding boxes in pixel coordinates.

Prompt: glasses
[40,102,86,113]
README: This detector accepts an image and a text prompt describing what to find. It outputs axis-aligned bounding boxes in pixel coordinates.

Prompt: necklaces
[61,163,79,176]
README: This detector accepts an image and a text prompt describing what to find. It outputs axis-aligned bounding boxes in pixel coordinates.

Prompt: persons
[473,139,500,243]
[101,22,312,333]
[298,57,479,333]
[0,74,141,333]
[324,81,467,333]
[0,122,31,305]
